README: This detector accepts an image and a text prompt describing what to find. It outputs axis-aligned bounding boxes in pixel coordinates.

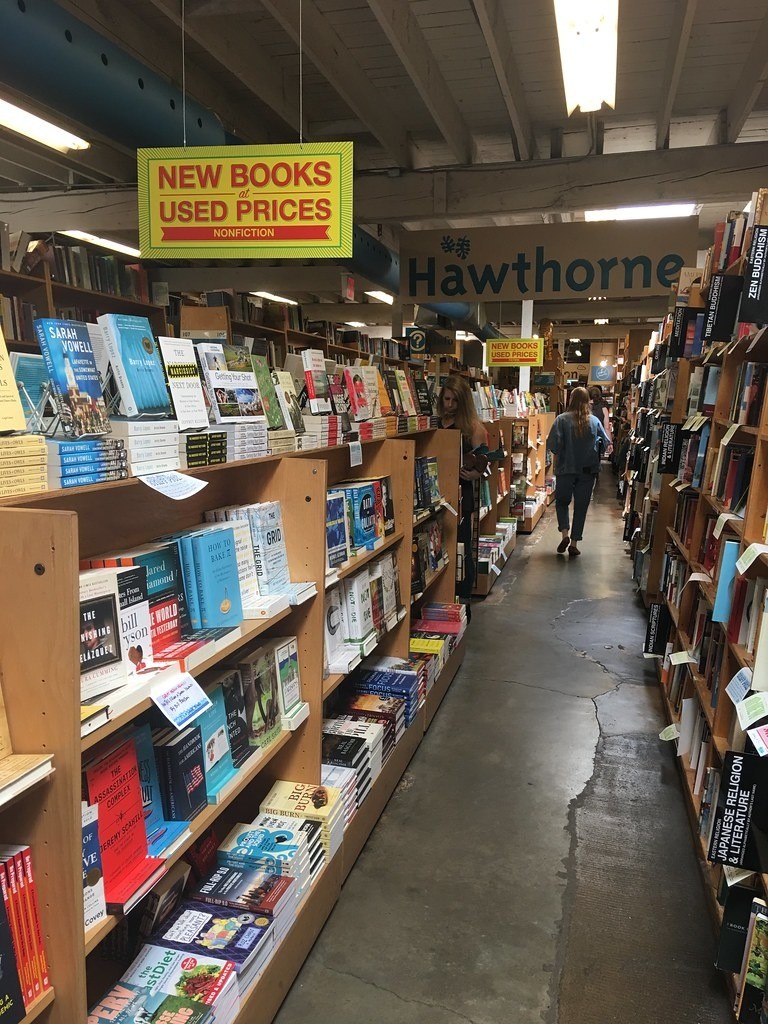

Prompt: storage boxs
[178,306,234,346]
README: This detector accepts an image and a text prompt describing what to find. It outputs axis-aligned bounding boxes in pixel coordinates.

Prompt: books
[614,188,768,1024]
[0,233,554,1024]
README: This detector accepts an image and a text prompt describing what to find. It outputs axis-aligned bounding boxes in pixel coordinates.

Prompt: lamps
[575,346,585,358]
[554,0,620,120]
[599,354,614,368]
[0,90,92,156]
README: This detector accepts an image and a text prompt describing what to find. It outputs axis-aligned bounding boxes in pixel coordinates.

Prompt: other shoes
[557,536,570,554]
[460,599,472,625]
[568,546,580,555]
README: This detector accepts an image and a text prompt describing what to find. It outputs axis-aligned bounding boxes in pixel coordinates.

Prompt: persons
[432,377,488,626]
[546,384,614,554]
[80,613,115,670]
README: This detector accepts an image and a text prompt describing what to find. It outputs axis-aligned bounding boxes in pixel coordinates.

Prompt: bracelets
[609,442,614,444]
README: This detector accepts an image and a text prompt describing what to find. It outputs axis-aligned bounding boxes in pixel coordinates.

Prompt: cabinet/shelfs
[0,260,569,1024]
[612,286,768,1024]
